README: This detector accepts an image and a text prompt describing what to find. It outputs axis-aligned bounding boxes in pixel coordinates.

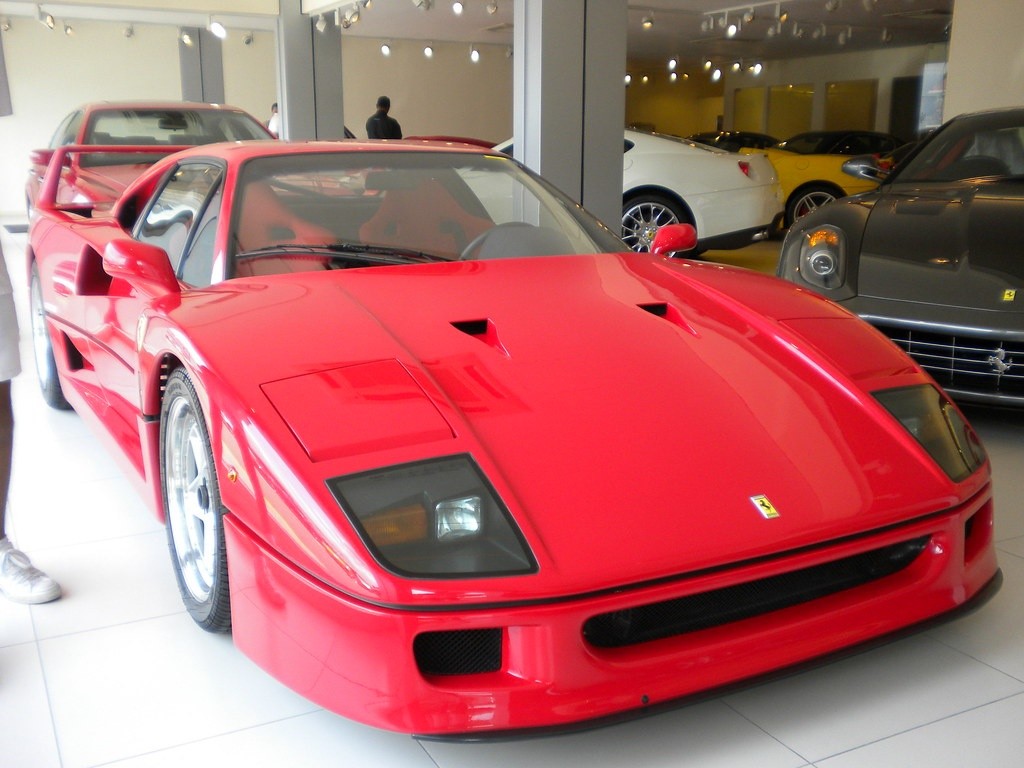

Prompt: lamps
[486,1,498,14]
[470,49,480,62]
[176,26,191,46]
[701,15,714,32]
[413,0,431,10]
[379,41,390,56]
[718,11,728,28]
[61,19,72,35]
[505,44,512,58]
[766,0,893,46]
[334,0,370,28]
[123,22,134,38]
[2,16,11,32]
[315,14,327,32]
[423,44,433,56]
[743,7,755,23]
[242,29,254,45]
[35,3,55,29]
[206,14,226,39]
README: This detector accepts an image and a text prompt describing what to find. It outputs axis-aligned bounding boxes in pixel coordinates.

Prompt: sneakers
[0,538,61,605]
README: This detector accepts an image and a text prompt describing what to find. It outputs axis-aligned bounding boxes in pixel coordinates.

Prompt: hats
[378,96,390,107]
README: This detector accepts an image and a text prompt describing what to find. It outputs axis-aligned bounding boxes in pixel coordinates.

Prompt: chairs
[359,176,496,266]
[938,154,1012,182]
[233,179,336,279]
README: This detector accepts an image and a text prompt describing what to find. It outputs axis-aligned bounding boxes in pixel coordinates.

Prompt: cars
[684,130,901,232]
[23,100,376,223]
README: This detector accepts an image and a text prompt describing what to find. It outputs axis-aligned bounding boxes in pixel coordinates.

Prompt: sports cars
[777,106,1023,401]
[27,139,1004,738]
[491,127,777,257]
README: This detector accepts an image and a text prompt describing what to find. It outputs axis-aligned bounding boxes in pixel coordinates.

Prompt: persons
[262,102,278,133]
[0,239,65,603]
[366,96,402,139]
